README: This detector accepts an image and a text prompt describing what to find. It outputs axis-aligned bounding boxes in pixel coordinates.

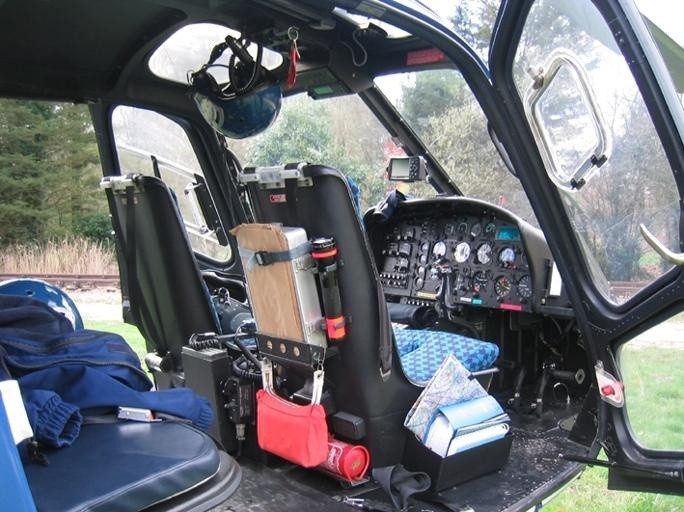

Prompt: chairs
[101,161,502,474]
[0,276,244,512]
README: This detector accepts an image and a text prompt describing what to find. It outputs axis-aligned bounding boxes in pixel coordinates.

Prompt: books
[423,395,511,458]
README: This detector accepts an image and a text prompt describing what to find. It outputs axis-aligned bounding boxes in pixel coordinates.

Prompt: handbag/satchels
[255,387,328,468]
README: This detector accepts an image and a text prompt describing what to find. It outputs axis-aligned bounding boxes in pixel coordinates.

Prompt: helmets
[188,35,284,140]
[3,274,85,335]
